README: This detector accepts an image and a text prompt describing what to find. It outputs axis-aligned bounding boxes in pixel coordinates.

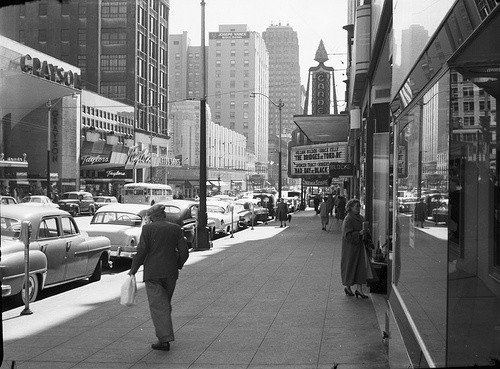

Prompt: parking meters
[19,220,35,315]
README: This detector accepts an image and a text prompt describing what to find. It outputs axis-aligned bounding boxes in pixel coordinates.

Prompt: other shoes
[152,342,170,351]
[322,228,326,231]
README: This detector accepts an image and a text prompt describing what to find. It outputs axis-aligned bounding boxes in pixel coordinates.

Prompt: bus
[122,184,174,206]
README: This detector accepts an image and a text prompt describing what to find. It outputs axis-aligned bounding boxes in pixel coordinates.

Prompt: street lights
[250,91,285,198]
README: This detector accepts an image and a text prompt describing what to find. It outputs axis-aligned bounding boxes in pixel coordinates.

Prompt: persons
[319,197,331,231]
[128,204,189,351]
[341,199,372,299]
[314,195,320,211]
[278,198,289,228]
[328,195,346,222]
[415,198,426,228]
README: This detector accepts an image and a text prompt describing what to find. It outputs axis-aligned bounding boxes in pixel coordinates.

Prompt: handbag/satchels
[120,273,138,306]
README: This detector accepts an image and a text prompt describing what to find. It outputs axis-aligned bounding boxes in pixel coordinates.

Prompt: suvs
[57,192,96,218]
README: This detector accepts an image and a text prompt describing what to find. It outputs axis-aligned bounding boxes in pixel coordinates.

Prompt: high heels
[344,287,354,296]
[355,290,368,298]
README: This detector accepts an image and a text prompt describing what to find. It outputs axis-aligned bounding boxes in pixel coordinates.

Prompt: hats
[147,204,165,216]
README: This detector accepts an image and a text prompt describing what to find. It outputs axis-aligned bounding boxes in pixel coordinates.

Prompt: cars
[205,188,300,237]
[21,196,60,209]
[85,204,153,268]
[0,202,112,305]
[152,200,216,248]
[91,196,119,212]
[0,196,18,204]
